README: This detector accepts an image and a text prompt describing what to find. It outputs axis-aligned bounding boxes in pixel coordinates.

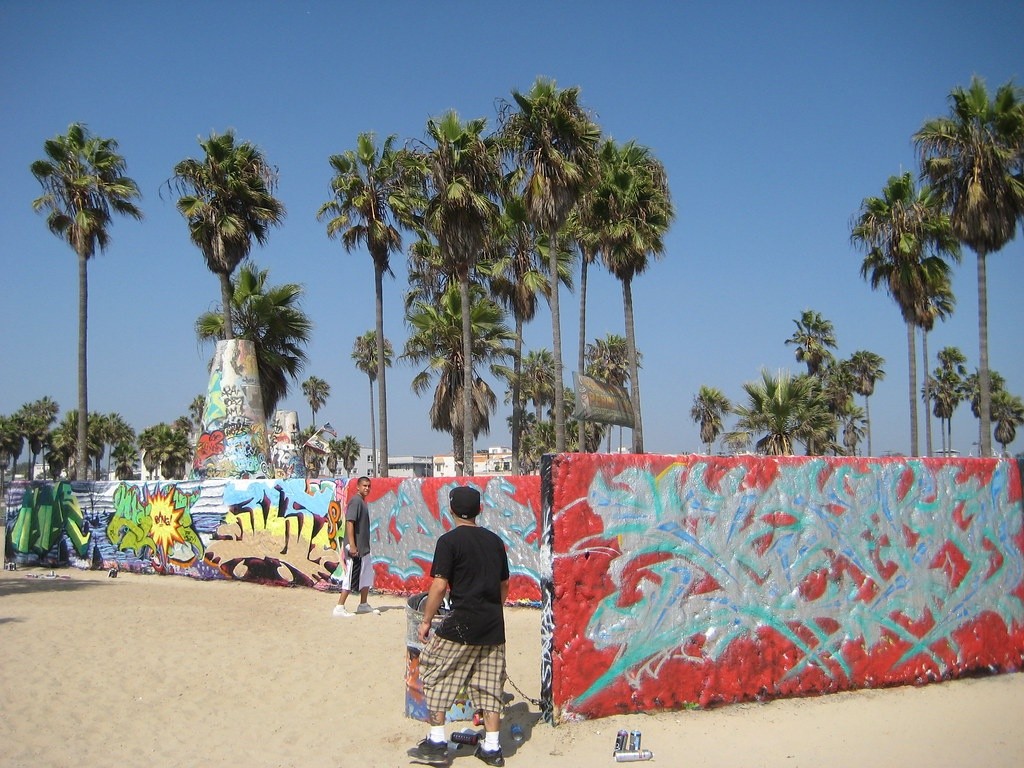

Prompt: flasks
[615,749,653,762]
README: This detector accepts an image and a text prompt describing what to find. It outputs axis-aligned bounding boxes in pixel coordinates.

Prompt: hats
[449,485,480,519]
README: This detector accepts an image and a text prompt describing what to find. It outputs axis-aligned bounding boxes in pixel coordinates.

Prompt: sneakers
[332,607,356,618]
[473,742,505,767]
[406,735,450,767]
[357,604,380,615]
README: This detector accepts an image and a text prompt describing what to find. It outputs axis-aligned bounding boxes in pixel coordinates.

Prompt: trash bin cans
[404,589,476,725]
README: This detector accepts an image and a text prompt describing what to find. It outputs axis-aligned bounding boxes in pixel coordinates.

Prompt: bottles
[511,723,524,741]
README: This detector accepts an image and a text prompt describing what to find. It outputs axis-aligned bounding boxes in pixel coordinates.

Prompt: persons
[406,487,510,767]
[332,476,380,617]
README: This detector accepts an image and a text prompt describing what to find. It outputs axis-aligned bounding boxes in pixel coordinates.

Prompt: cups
[451,732,478,745]
[447,740,463,750]
[613,730,628,757]
[629,730,641,751]
[461,727,482,740]
